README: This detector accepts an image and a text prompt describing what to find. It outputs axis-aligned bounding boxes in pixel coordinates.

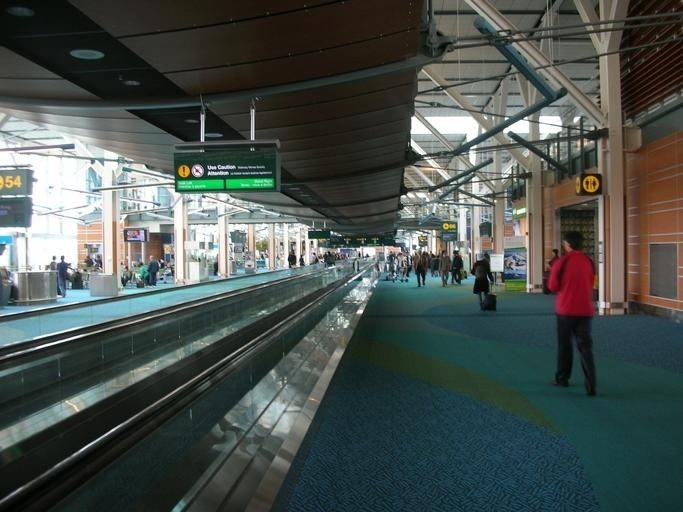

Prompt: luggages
[136,275,144,288]
[483,284,496,311]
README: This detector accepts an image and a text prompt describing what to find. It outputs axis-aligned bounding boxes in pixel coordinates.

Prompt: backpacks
[460,271,467,280]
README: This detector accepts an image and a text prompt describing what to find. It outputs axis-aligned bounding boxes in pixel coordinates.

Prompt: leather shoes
[551,379,568,385]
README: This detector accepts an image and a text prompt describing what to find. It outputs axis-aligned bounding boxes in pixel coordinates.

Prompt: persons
[49,255,59,294]
[470,251,494,312]
[56,255,68,297]
[382,248,466,288]
[83,254,149,286]
[546,248,560,268]
[287,249,296,269]
[146,255,159,287]
[298,250,347,268]
[545,230,599,395]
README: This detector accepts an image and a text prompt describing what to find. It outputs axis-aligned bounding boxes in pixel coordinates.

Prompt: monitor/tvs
[123,227,150,242]
